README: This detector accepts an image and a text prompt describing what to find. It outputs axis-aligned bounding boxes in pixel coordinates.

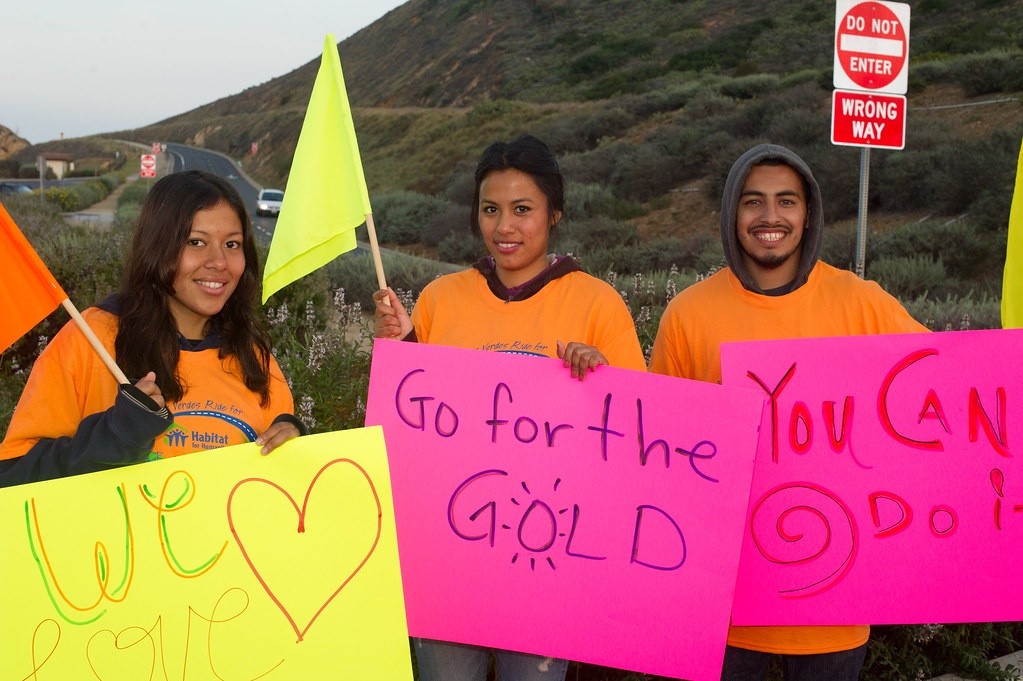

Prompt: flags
[262,34,372,305]
[0,202,66,352]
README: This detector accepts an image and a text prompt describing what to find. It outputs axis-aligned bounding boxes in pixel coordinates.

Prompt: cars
[256,187,284,218]
[0,180,35,198]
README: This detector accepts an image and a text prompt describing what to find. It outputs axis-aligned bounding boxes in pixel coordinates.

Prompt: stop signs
[833,0,910,96]
[141,154,156,171]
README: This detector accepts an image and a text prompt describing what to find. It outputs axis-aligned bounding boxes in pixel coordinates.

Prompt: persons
[375,134,647,681]
[0,171,304,493]
[646,142,937,681]
